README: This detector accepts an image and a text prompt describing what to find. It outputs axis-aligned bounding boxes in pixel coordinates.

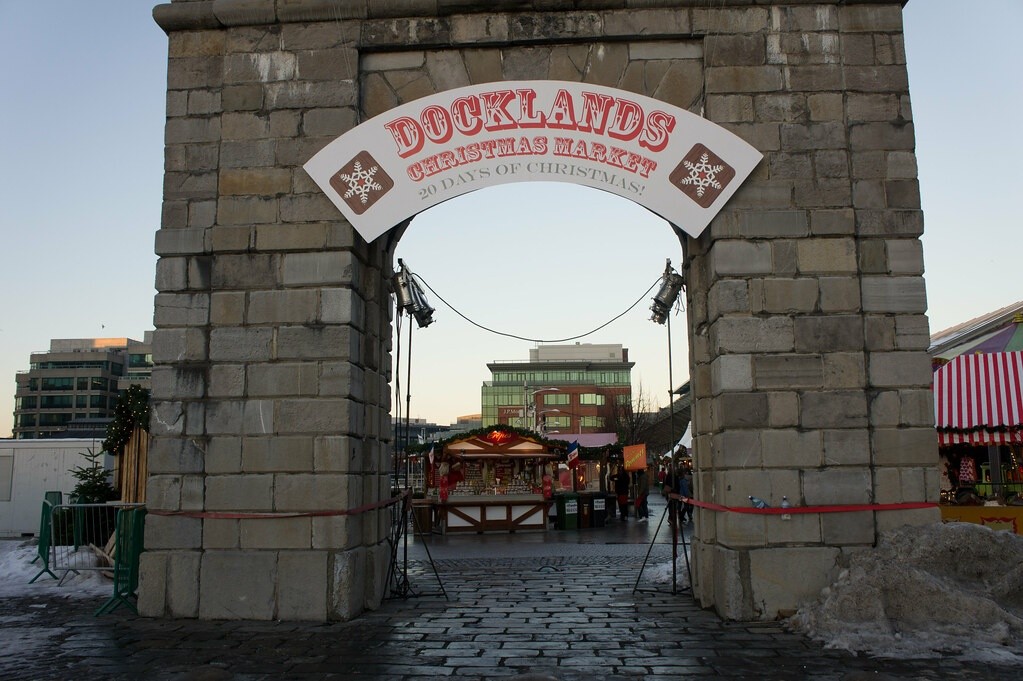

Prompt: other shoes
[637,517,648,523]
[668,519,673,524]
[683,517,687,521]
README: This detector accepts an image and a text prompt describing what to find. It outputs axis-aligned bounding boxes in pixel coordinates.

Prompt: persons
[635,469,649,522]
[610,466,630,521]
[659,464,693,525]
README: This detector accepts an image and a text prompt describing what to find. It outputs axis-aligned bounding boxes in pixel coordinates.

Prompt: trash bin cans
[412,499,434,537]
[588,492,606,528]
[554,492,578,530]
[578,496,590,529]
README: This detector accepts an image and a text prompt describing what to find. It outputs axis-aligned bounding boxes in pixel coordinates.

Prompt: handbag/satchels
[619,495,628,504]
[689,478,693,494]
[664,485,672,492]
[635,497,642,508]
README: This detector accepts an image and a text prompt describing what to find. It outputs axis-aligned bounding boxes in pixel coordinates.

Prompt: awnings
[933,312,1023,447]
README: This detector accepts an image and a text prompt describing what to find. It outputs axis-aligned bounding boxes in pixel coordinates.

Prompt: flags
[429,443,434,472]
[623,445,647,470]
[566,443,580,471]
